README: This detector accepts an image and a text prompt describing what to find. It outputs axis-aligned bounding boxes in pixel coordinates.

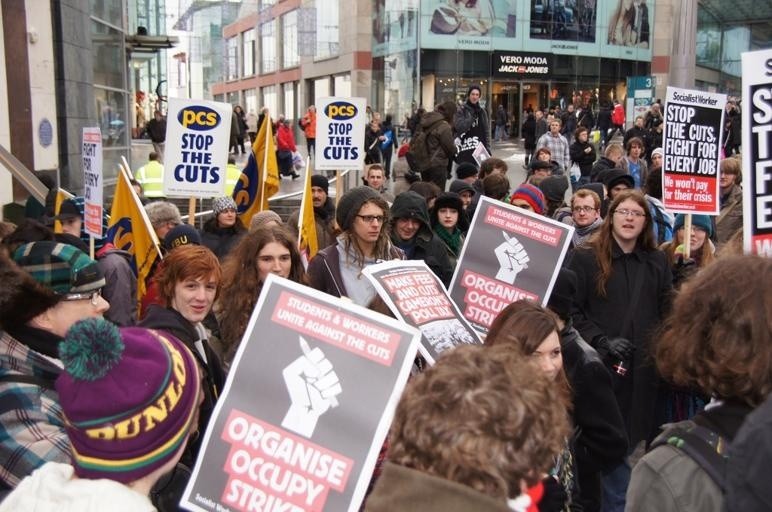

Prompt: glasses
[614,208,646,217]
[61,286,102,306]
[574,205,597,212]
[356,214,384,222]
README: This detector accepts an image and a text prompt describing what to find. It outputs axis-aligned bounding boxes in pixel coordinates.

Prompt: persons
[453,85,492,164]
[483,298,563,383]
[386,189,452,290]
[364,346,566,512]
[136,246,234,463]
[0,240,110,498]
[545,276,629,511]
[408,181,441,209]
[307,185,409,319]
[522,108,536,169]
[450,180,476,231]
[591,143,623,182]
[286,176,340,255]
[715,158,743,246]
[645,103,663,130]
[623,116,650,152]
[146,104,398,170]
[0,318,204,512]
[50,197,108,244]
[650,147,663,167]
[609,100,626,139]
[539,175,569,217]
[483,173,510,203]
[570,128,596,190]
[724,101,741,154]
[532,161,553,179]
[602,169,635,215]
[367,165,394,206]
[608,0,650,49]
[534,119,569,175]
[546,107,556,129]
[448,0,489,36]
[723,119,733,157]
[140,224,201,318]
[94,244,138,327]
[141,202,185,239]
[535,111,549,143]
[201,196,249,258]
[527,148,563,179]
[207,226,305,402]
[107,178,142,215]
[471,159,508,217]
[455,163,478,184]
[619,137,647,188]
[568,192,673,454]
[623,256,772,512]
[596,103,614,137]
[26,174,54,218]
[562,189,604,251]
[561,104,577,133]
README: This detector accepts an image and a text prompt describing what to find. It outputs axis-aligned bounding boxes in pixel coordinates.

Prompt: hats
[547,266,577,320]
[429,192,470,232]
[468,83,481,98]
[48,199,78,222]
[398,144,409,156]
[457,162,478,179]
[144,201,184,229]
[532,160,553,169]
[673,213,712,237]
[54,319,200,482]
[539,174,568,202]
[311,174,329,194]
[211,195,238,215]
[336,185,381,230]
[160,224,200,253]
[651,147,663,158]
[0,221,106,326]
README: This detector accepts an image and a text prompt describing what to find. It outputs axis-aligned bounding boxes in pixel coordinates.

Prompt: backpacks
[406,120,445,171]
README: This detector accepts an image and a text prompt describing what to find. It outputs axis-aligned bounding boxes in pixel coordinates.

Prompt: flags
[298,163,319,261]
[233,115,280,226]
[55,190,71,233]
[108,171,158,295]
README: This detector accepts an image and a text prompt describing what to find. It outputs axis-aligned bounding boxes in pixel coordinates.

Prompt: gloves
[597,335,636,363]
[671,252,696,290]
